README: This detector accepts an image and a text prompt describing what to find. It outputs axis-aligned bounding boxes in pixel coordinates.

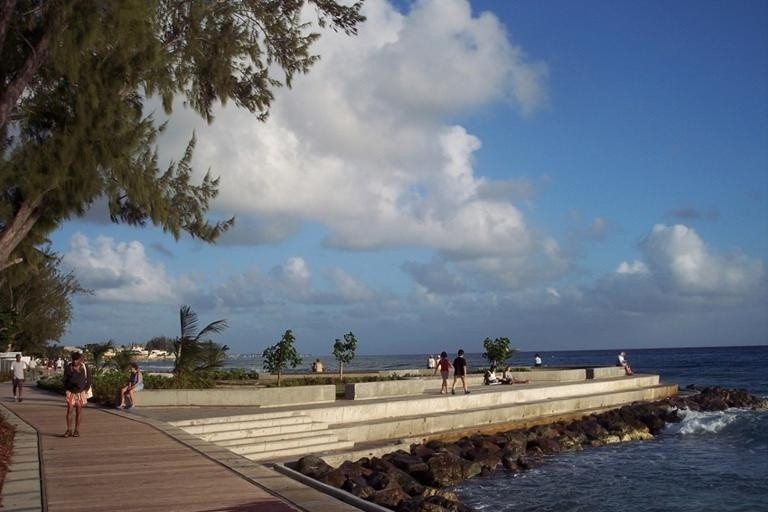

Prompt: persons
[533,353,542,367]
[483,365,513,386]
[62,353,94,438]
[451,349,470,394]
[116,362,144,409]
[427,355,435,369]
[312,358,323,373]
[435,354,442,369]
[433,352,453,394]
[504,366,532,384]
[10,351,27,402]
[616,351,634,375]
[29,354,62,382]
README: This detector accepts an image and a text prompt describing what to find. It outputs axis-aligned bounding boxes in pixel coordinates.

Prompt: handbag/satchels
[86,386,93,399]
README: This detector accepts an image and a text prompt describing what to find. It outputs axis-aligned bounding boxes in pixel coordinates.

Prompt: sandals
[117,406,135,410]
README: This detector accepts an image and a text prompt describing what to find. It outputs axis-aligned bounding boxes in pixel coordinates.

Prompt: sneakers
[441,390,455,394]
[63,430,80,438]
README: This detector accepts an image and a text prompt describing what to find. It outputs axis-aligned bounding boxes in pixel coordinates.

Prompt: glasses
[18,357,21,358]
